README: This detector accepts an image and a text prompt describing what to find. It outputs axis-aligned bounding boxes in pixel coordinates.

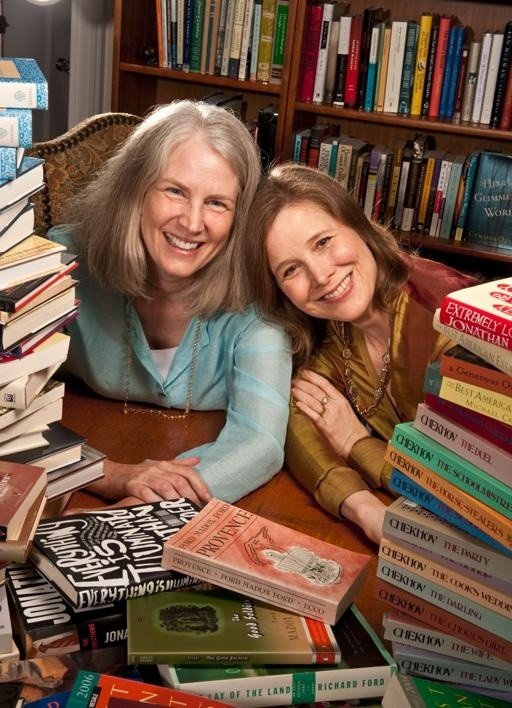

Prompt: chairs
[25,113,146,242]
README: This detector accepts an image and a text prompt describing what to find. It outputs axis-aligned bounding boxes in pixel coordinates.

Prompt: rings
[319,393,331,406]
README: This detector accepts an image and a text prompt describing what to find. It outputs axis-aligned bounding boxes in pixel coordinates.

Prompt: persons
[244,161,482,552]
[41,99,293,529]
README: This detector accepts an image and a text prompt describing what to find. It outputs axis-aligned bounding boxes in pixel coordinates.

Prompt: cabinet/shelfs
[110,0,299,174]
[280,0,512,282]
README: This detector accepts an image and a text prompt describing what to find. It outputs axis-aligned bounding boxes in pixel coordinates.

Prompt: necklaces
[121,291,206,421]
[336,320,397,420]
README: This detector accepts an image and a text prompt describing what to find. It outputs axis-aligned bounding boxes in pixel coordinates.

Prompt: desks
[59,379,394,663]
